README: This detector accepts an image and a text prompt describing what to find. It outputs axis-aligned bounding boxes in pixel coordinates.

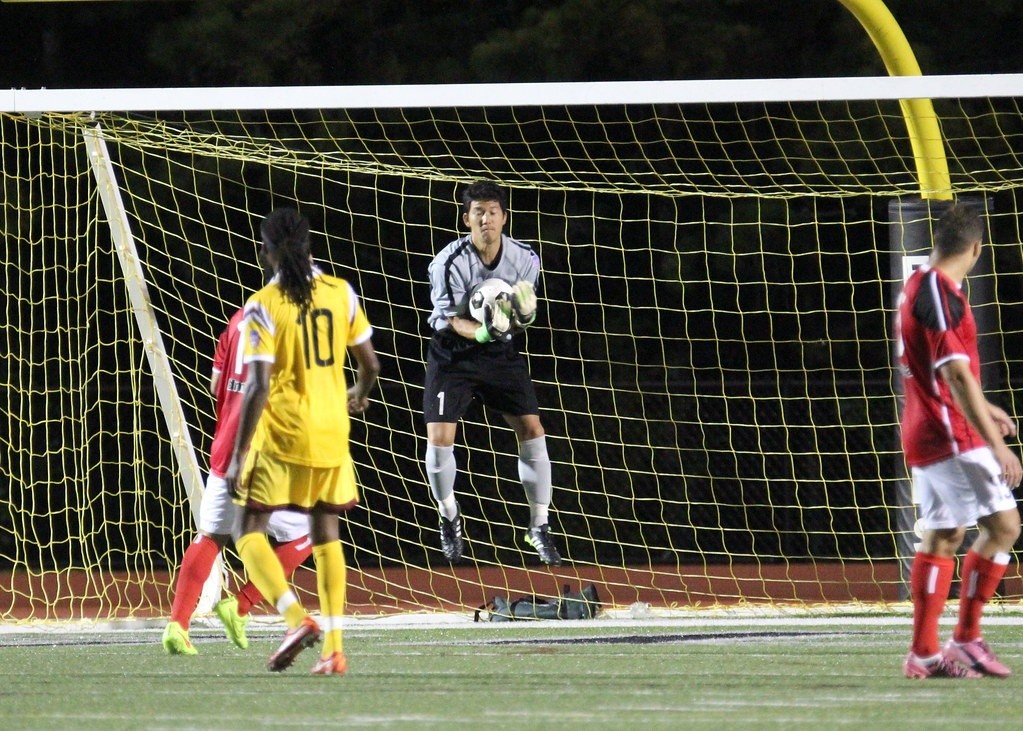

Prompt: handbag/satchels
[475,582,602,620]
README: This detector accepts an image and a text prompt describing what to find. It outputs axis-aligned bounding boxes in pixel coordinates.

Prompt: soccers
[468,278,513,323]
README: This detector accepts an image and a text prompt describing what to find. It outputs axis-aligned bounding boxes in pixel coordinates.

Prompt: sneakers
[214,595,251,649]
[266,618,321,672]
[162,622,197,656]
[437,498,463,562]
[903,649,983,679]
[942,636,1011,677]
[525,524,561,567]
[302,652,346,675]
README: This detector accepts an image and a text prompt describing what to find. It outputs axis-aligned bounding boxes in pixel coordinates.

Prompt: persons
[225,210,379,677]
[896,205,1023,680]
[423,181,561,565]
[161,309,311,653]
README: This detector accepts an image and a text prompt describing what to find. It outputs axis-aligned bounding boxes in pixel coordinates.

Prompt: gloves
[511,280,536,316]
[474,299,513,344]
